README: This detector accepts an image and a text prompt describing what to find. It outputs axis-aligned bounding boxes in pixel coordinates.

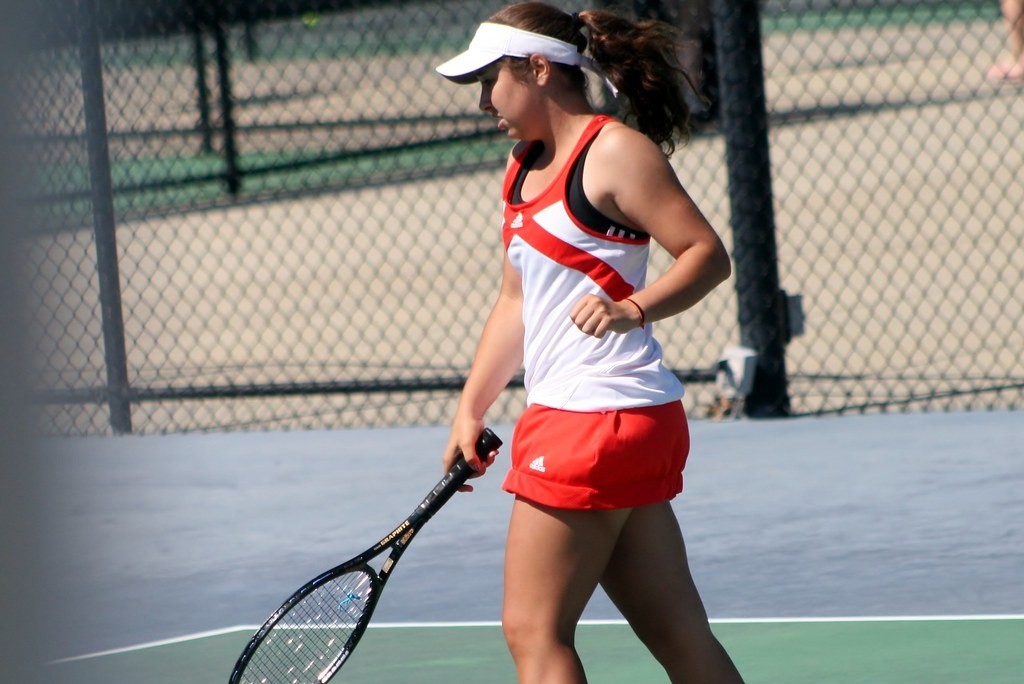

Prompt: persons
[434,0,745,684]
[985,1,1024,83]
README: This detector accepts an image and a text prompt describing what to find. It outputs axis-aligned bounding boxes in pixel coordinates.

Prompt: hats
[436,23,618,98]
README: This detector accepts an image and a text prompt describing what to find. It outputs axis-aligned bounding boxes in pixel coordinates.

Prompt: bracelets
[625,298,645,330]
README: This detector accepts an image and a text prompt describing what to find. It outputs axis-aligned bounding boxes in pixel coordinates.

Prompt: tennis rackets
[227,427,504,684]
